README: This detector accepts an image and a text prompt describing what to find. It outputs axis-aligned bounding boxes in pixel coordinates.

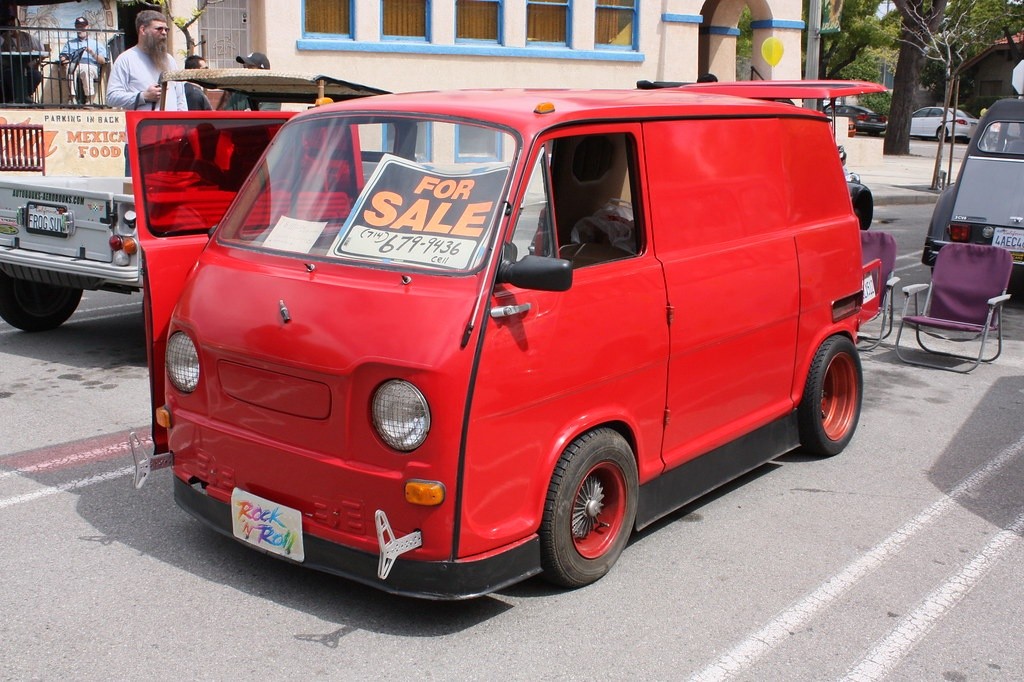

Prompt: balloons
[762,37,784,67]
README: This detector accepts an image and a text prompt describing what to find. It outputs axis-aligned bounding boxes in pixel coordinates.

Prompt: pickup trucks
[0,65,393,331]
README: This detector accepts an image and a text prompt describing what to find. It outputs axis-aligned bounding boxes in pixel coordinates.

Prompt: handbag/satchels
[571,198,636,256]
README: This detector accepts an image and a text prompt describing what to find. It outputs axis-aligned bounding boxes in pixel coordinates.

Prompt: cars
[920,96,1024,310]
[823,104,888,136]
[910,106,980,143]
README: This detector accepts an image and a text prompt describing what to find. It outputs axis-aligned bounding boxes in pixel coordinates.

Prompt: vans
[124,75,885,602]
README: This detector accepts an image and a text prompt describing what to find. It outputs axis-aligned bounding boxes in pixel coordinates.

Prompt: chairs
[856,231,902,351]
[77,53,111,107]
[895,243,1014,373]
[560,215,635,270]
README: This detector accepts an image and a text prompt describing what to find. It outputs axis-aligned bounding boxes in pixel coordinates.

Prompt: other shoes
[68,99,77,105]
[83,101,93,108]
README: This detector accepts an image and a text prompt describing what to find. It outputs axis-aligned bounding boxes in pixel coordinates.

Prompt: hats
[75,17,88,25]
[236,53,270,69]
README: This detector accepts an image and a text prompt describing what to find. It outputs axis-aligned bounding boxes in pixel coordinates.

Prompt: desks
[38,60,72,103]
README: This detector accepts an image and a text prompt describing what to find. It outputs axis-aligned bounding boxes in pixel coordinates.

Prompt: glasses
[155,27,170,34]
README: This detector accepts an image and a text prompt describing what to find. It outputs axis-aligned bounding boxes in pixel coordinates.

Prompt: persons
[221,53,282,111]
[59,16,105,104]
[105,11,190,179]
[182,56,215,111]
